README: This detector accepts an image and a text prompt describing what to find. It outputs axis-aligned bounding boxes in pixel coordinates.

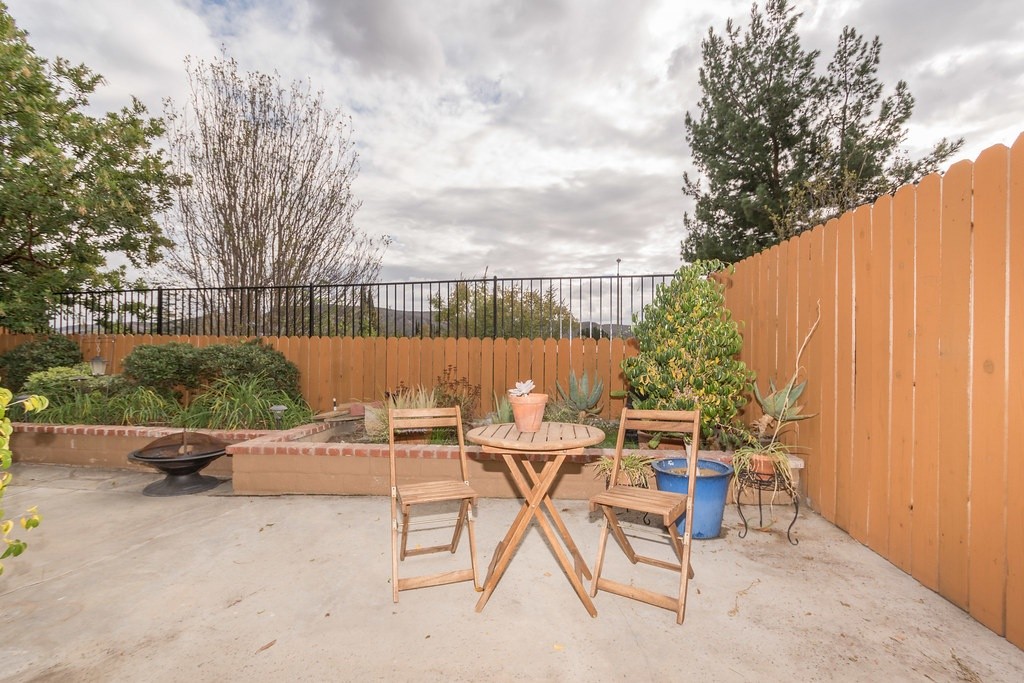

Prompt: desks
[465,419,596,616]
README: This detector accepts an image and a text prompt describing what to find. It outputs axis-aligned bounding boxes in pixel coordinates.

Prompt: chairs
[386,405,486,606]
[587,401,707,623]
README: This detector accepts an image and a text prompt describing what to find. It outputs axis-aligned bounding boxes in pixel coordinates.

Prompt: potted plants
[721,363,822,486]
[620,257,755,539]
[505,378,550,432]
